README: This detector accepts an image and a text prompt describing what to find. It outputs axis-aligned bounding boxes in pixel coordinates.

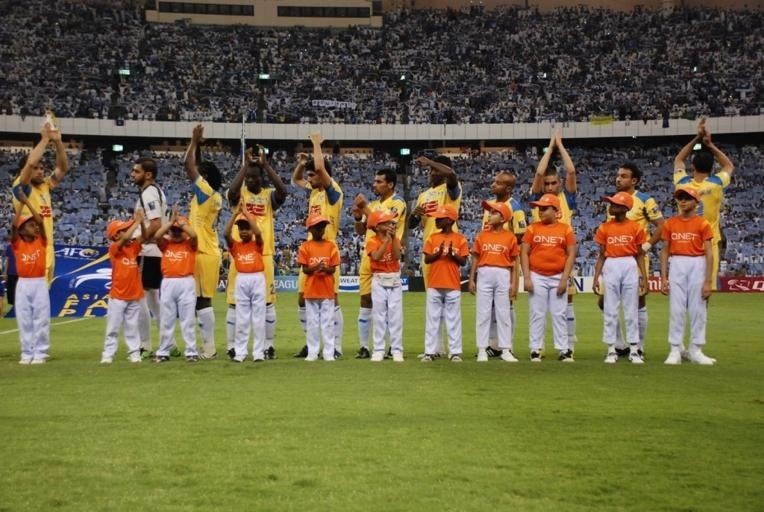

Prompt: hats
[15,215,34,230]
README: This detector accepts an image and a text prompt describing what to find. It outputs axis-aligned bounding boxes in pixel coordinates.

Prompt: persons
[598,160,667,360]
[672,116,735,363]
[473,171,529,360]
[225,141,289,361]
[221,195,267,363]
[98,207,149,365]
[659,187,716,367]
[349,168,407,360]
[467,201,521,364]
[405,155,463,359]
[1,0,763,313]
[183,123,225,361]
[290,127,343,359]
[149,203,198,362]
[519,193,578,363]
[11,122,70,359]
[365,209,405,363]
[591,190,650,365]
[10,184,51,365]
[126,156,181,362]
[525,126,578,361]
[421,205,469,364]
[295,212,342,362]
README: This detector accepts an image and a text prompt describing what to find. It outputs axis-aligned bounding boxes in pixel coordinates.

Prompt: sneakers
[32,359,46,364]
[19,359,31,365]
